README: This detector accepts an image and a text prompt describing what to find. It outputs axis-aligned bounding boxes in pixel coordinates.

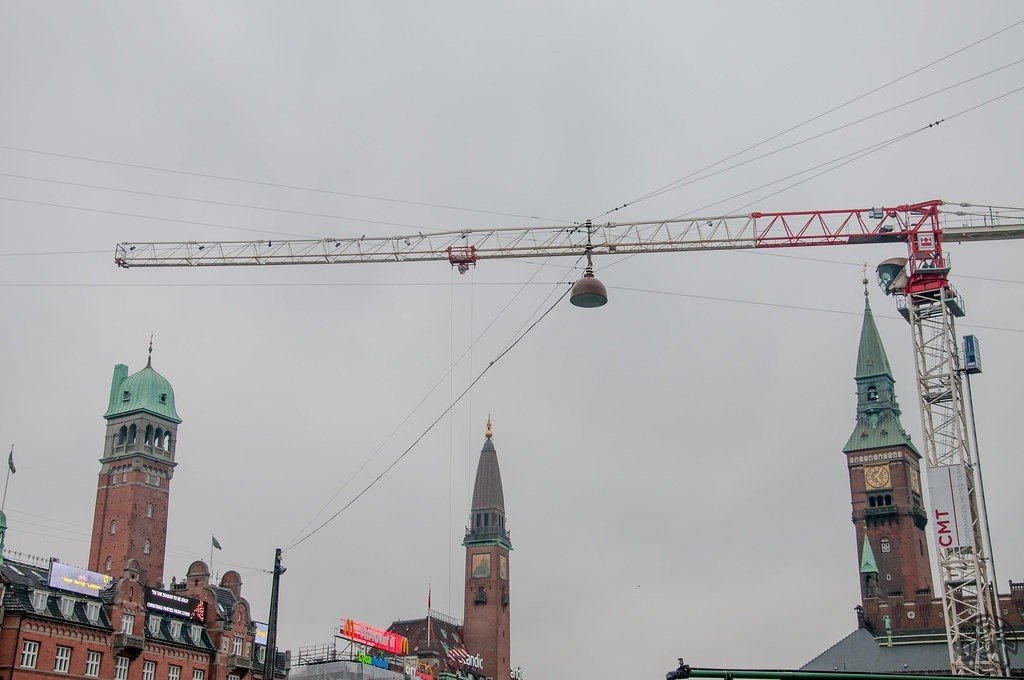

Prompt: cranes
[114,196,1023,677]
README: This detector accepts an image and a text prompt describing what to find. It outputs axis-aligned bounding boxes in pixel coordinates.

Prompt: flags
[212,537,221,551]
[8,450,16,474]
